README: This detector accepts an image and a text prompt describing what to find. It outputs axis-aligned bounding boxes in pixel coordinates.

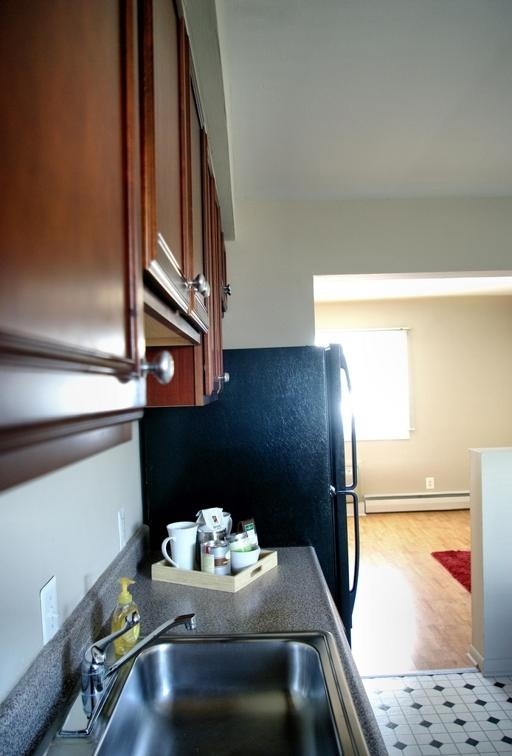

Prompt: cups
[161,512,260,578]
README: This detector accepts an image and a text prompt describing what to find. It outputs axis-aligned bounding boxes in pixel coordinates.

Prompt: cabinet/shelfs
[142,175,231,413]
[137,0,210,358]
[2,4,174,495]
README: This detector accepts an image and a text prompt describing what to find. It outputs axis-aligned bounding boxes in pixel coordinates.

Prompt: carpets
[430,549,471,595]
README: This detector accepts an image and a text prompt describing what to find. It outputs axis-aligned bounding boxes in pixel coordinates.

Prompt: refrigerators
[135,344,360,657]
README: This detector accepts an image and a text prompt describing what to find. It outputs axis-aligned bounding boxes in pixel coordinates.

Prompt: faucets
[80,610,196,716]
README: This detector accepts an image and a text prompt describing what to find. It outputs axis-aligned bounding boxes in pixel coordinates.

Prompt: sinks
[34,631,371,755]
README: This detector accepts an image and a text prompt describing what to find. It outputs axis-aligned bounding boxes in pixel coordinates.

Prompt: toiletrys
[111,578,141,655]
